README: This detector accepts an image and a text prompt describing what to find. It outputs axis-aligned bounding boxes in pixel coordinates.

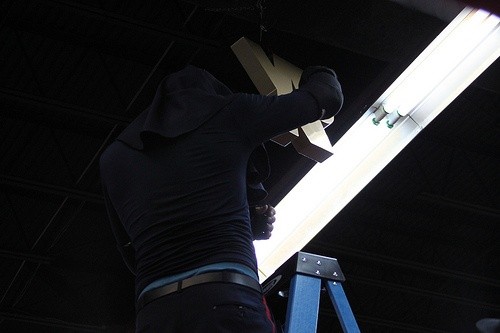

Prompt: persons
[91,57,344,333]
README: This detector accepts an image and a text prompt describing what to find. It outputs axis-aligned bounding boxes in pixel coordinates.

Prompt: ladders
[283,252,361,333]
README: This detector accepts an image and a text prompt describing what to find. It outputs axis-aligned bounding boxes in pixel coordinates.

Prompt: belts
[133,270,264,315]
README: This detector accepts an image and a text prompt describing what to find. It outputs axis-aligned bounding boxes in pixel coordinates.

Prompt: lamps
[253,8,500,286]
